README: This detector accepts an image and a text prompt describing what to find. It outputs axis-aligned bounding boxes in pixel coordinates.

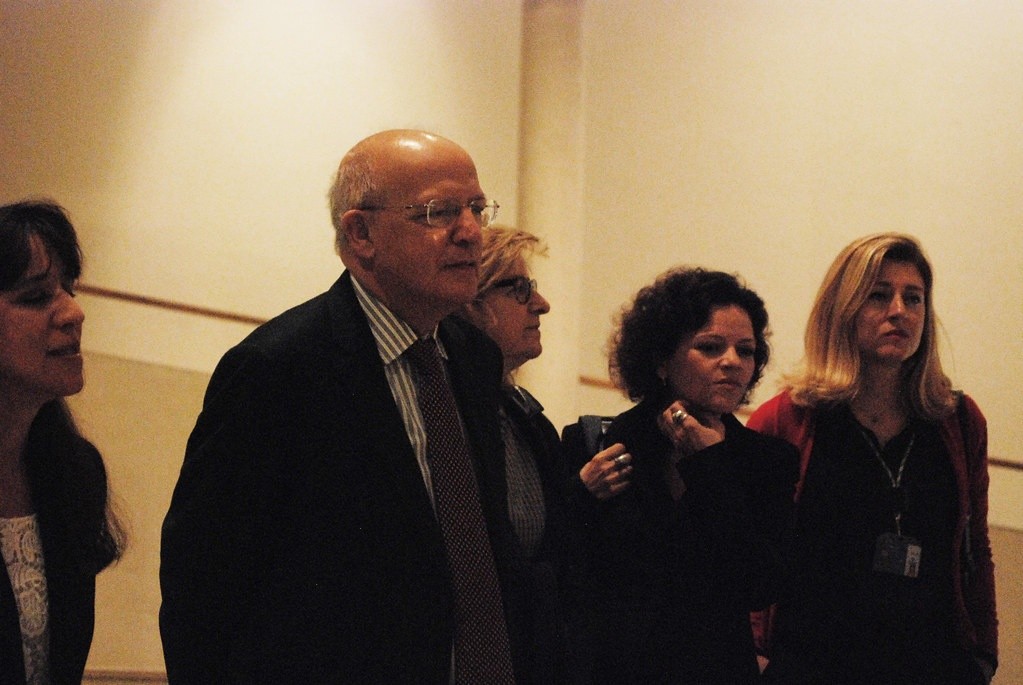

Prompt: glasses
[495,275,538,304]
[358,197,499,228]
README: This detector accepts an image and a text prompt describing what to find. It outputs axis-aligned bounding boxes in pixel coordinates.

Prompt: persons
[0,197,136,685]
[436,224,570,684]
[745,232,999,685]
[561,263,801,685]
[158,128,516,684]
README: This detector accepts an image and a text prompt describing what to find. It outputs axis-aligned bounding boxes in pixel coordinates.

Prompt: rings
[614,456,627,470]
[672,410,688,425]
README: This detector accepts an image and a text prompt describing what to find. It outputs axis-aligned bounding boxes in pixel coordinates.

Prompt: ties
[404,339,514,685]
